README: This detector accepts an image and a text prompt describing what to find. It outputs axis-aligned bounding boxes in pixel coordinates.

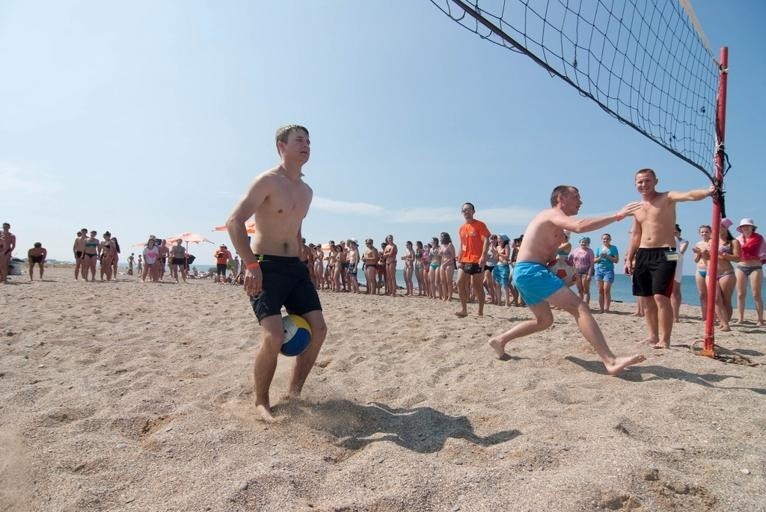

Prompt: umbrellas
[137,232,215,269]
[213,221,256,234]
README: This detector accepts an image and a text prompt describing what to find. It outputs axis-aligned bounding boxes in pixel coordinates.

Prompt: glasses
[462,208,473,212]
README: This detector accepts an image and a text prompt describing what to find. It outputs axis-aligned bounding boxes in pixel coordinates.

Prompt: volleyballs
[279,315,313,357]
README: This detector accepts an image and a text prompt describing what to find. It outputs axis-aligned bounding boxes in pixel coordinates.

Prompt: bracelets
[614,212,623,221]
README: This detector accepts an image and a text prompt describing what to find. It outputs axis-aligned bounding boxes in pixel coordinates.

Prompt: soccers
[549,259,580,287]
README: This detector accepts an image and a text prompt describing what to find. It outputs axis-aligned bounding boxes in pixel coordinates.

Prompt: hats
[736,218,758,235]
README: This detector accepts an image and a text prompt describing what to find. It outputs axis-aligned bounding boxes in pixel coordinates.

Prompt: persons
[486,186,647,376]
[0,223,15,282]
[453,202,490,318]
[225,125,327,424]
[569,235,594,307]
[623,222,689,323]
[27,242,46,281]
[592,233,619,314]
[692,225,713,320]
[735,218,766,327]
[127,234,190,285]
[624,168,715,348]
[72,229,120,281]
[714,218,741,333]
